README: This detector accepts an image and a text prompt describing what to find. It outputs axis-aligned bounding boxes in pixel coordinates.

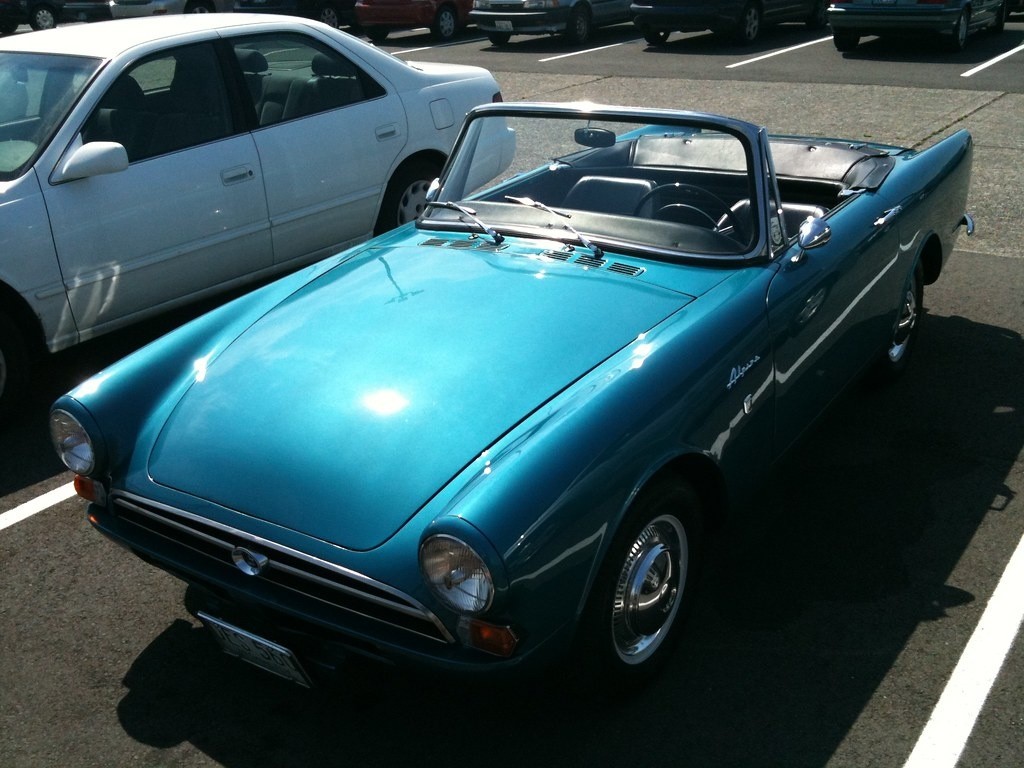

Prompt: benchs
[233,47,363,128]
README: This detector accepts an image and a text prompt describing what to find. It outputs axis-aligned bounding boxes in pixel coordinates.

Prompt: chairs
[146,69,224,160]
[711,198,831,246]
[79,74,160,161]
[555,175,663,223]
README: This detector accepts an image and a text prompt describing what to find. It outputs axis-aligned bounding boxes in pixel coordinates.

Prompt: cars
[467,0,631,46]
[629,0,829,44]
[232,0,354,29]
[353,0,473,42]
[48,98,974,689]
[0,0,65,36]
[62,0,235,24]
[0,13,516,419]
[826,0,1007,52]
